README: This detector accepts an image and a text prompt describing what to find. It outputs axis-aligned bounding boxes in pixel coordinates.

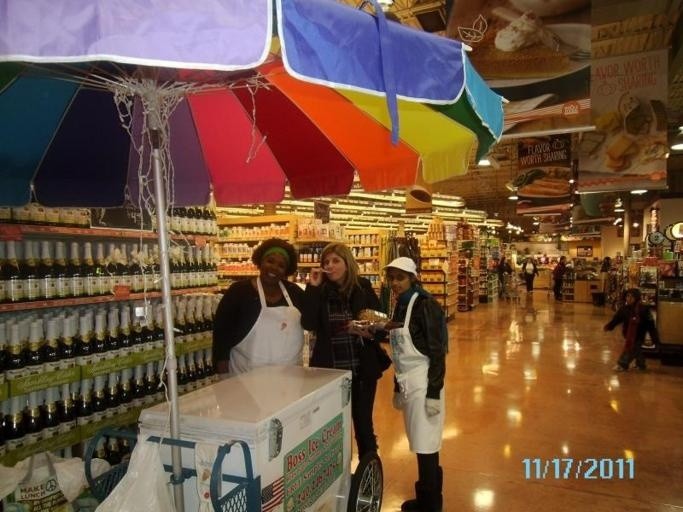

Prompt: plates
[517,166,571,199]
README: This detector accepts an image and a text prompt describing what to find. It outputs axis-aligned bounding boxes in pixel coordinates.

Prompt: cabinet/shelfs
[217,215,518,320]
[558,273,575,303]
[607,251,682,368]
[0,224,221,512]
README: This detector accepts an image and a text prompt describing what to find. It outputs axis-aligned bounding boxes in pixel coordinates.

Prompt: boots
[401,467,443,511]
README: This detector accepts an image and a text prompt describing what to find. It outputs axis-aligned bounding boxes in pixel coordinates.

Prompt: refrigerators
[134,361,353,511]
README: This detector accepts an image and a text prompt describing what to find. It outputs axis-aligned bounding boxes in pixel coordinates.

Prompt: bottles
[221,223,289,255]
[0,290,224,467]
[0,241,218,303]
[0,198,218,236]
[299,250,319,263]
[221,258,259,273]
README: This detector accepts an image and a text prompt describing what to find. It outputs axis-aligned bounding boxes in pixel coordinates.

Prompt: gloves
[393,393,403,411]
[426,398,441,416]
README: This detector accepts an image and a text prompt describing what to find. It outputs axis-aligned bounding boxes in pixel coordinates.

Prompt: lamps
[505,142,519,201]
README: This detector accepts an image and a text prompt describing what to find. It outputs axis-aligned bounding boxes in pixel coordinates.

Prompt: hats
[384,257,418,277]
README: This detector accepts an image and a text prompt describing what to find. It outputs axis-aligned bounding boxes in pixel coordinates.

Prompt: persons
[522,258,539,292]
[600,256,611,292]
[304,243,393,459]
[213,238,311,380]
[554,255,568,300]
[364,255,447,512]
[603,288,660,372]
[498,256,512,297]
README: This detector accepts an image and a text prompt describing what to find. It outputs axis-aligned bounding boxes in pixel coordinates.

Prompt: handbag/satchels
[14,452,72,512]
[356,341,392,376]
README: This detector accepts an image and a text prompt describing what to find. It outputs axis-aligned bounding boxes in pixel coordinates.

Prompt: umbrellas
[0,1,510,512]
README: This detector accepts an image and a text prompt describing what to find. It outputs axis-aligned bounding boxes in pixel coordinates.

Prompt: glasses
[388,275,405,280]
